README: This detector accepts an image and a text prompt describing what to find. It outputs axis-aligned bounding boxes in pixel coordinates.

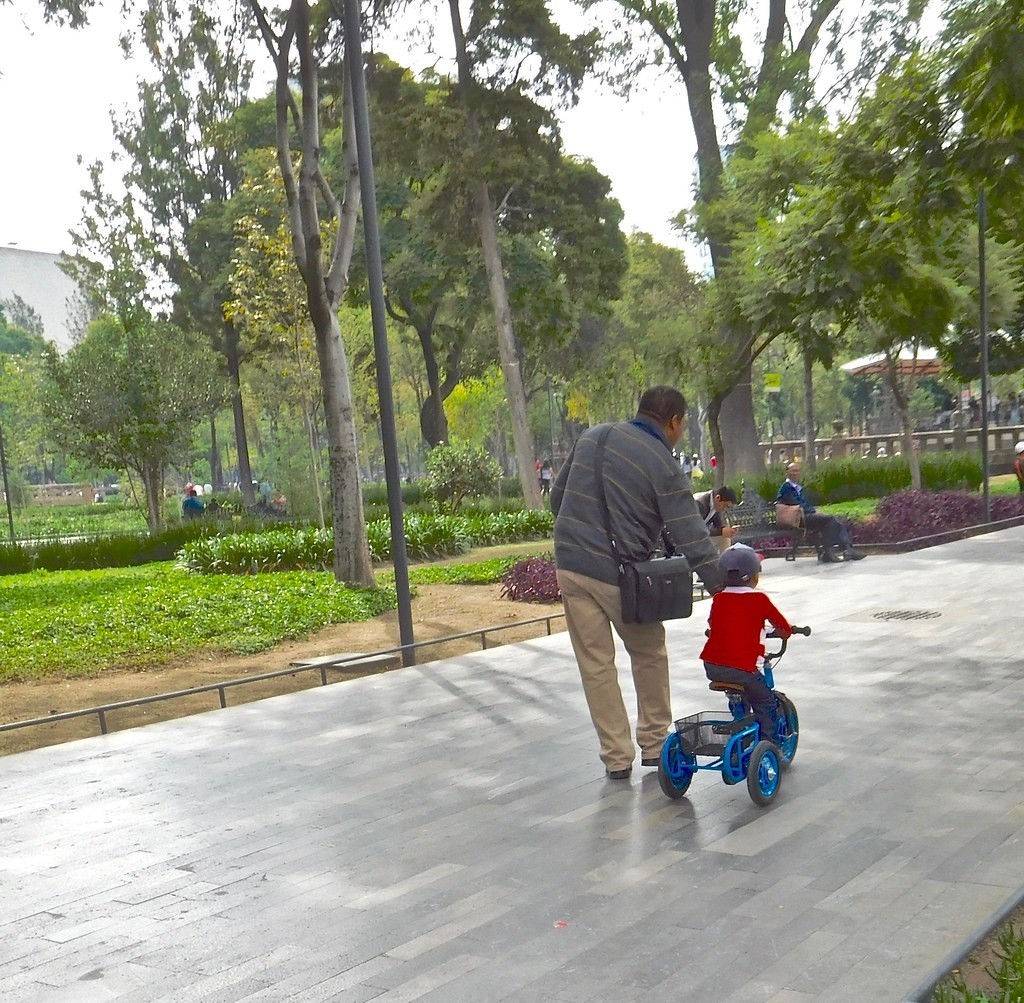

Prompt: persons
[549,385,720,778]
[182,478,286,519]
[692,486,739,552]
[777,462,866,562]
[700,543,792,762]
[535,458,554,495]
[940,388,1024,491]
[674,450,718,479]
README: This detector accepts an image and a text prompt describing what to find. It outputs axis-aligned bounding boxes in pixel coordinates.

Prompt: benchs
[724,479,823,561]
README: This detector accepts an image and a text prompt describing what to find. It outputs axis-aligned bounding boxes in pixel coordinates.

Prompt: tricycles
[656,626,812,807]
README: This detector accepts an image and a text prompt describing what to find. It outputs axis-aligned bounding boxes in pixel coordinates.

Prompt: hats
[716,542,765,585]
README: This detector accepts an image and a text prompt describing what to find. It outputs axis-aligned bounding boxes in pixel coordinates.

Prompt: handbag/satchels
[775,504,806,537]
[618,553,693,625]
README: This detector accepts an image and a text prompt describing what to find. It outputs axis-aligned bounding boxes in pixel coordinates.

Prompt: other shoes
[642,758,659,765]
[843,549,867,560]
[822,553,844,563]
[606,765,632,779]
